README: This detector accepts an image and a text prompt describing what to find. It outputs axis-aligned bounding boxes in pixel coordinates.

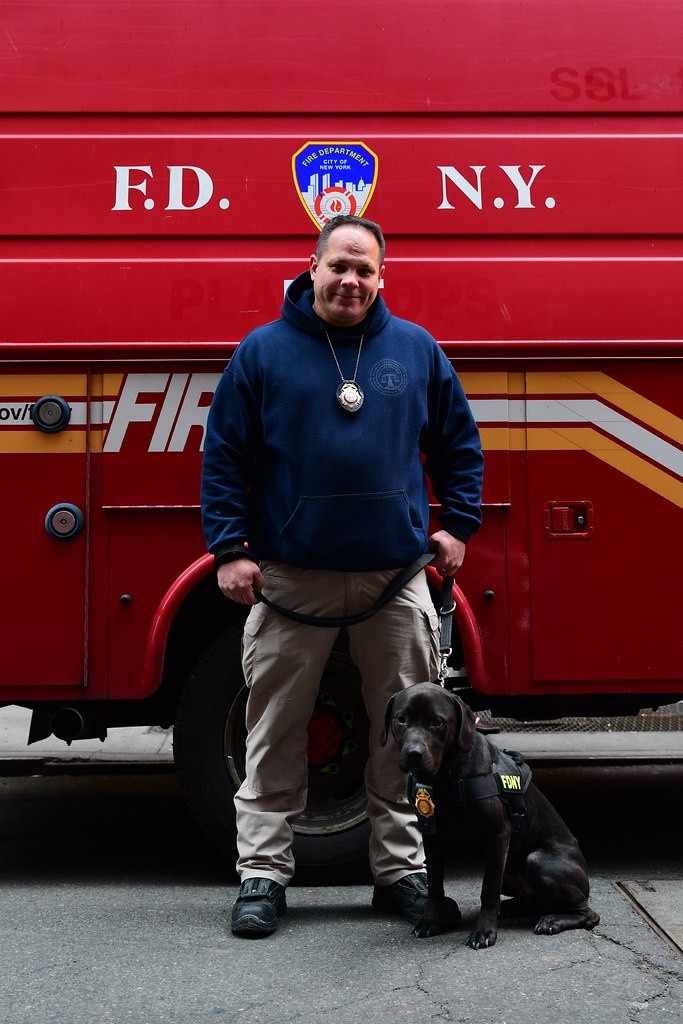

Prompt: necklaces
[325,330,364,413]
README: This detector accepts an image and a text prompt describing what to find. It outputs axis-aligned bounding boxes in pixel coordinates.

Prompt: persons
[197,216,486,938]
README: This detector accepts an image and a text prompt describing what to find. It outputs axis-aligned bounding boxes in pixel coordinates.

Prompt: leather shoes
[370,872,463,929]
[231,877,289,935]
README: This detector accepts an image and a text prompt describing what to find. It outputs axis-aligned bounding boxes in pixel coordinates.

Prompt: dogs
[380,681,600,950]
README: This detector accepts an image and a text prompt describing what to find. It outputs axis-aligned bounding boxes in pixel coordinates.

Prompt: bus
[0,1,682,886]
